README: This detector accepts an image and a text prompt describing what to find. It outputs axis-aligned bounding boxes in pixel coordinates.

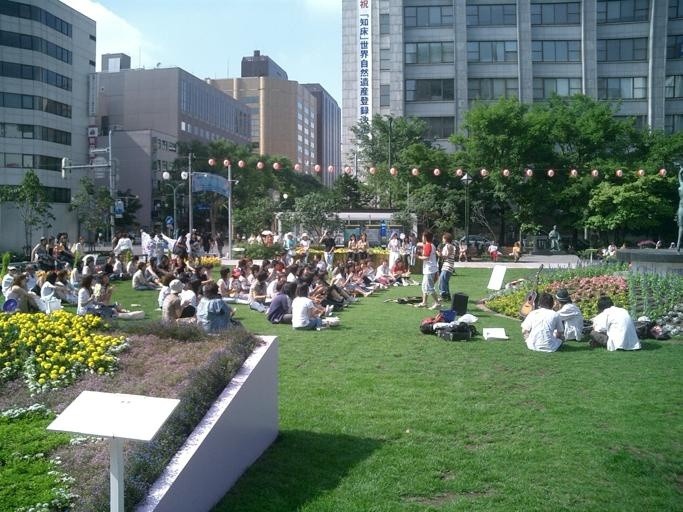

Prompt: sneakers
[415,303,427,309]
[403,281,420,287]
[438,296,451,302]
[363,284,380,297]
[337,296,358,303]
[324,304,335,317]
[427,304,441,311]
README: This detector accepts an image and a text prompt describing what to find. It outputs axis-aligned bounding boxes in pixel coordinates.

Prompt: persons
[548,224,562,254]
[519,292,565,354]
[457,235,499,263]
[512,240,521,263]
[552,288,585,342]
[674,166,682,252]
[608,240,618,257]
[0,225,455,334]
[588,296,642,353]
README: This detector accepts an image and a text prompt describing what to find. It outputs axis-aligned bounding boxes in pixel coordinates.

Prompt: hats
[98,272,109,279]
[300,233,310,240]
[7,265,17,270]
[232,268,243,277]
[284,232,296,239]
[168,279,186,294]
[555,288,571,302]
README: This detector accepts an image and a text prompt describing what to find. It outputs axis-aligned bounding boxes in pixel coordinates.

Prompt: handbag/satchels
[118,310,145,320]
[173,241,186,257]
[325,317,339,327]
[422,317,432,326]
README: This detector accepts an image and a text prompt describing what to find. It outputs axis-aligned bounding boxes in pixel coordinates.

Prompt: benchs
[482,247,521,261]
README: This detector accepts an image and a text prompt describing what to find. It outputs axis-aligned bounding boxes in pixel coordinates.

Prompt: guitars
[518,264,544,319]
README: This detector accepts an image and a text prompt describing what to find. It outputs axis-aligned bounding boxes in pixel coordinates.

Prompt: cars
[460,235,499,250]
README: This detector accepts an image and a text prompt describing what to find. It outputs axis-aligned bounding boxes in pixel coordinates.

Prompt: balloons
[206,156,670,179]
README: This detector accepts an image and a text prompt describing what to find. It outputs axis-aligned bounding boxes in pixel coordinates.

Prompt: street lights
[460,172,472,239]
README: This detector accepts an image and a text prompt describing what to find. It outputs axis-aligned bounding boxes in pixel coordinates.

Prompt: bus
[273,212,417,246]
